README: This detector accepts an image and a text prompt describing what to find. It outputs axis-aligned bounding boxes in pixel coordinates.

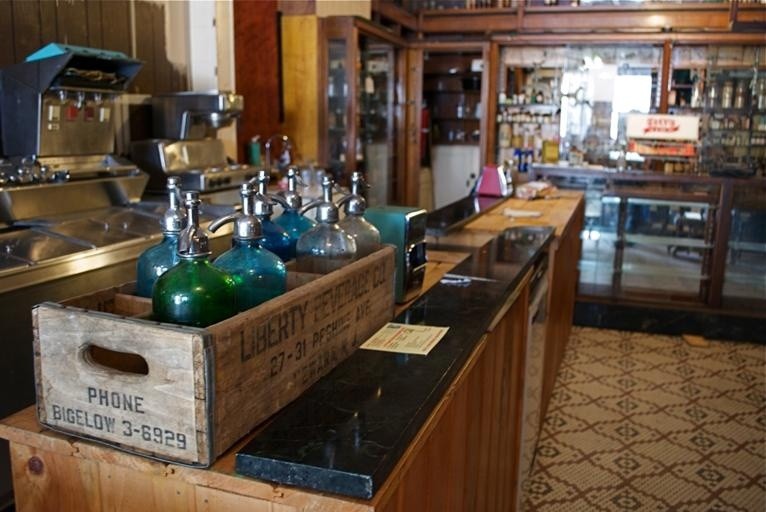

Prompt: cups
[0,152,71,191]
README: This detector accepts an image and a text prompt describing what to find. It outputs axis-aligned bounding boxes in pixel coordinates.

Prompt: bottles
[329,62,376,162]
[446,93,469,142]
[688,74,766,112]
[495,88,560,125]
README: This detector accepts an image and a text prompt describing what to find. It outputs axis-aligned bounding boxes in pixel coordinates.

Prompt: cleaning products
[139,162,382,324]
